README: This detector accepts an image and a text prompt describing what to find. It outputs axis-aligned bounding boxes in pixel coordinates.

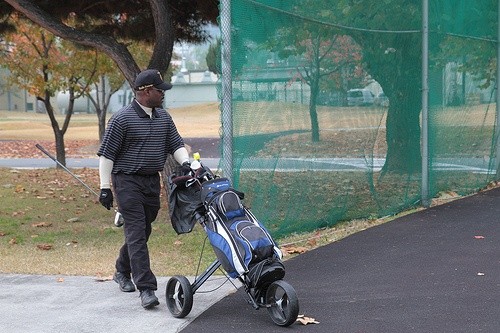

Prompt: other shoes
[113,270,135,292]
[140,289,158,308]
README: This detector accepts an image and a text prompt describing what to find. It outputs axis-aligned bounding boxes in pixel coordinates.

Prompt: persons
[97,70,194,308]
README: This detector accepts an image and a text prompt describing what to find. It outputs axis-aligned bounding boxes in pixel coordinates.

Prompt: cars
[316,86,389,107]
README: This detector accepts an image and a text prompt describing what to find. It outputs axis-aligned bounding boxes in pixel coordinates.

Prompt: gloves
[99,188,113,210]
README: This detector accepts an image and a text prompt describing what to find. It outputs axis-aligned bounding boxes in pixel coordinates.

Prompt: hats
[133,70,172,91]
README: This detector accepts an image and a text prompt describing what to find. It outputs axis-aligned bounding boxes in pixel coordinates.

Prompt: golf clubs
[185,160,214,187]
[35,143,124,227]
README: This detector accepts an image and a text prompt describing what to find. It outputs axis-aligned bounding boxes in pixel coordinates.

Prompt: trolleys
[163,152,300,327]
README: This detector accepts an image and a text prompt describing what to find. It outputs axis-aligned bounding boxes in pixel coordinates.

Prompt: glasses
[155,89,165,93]
